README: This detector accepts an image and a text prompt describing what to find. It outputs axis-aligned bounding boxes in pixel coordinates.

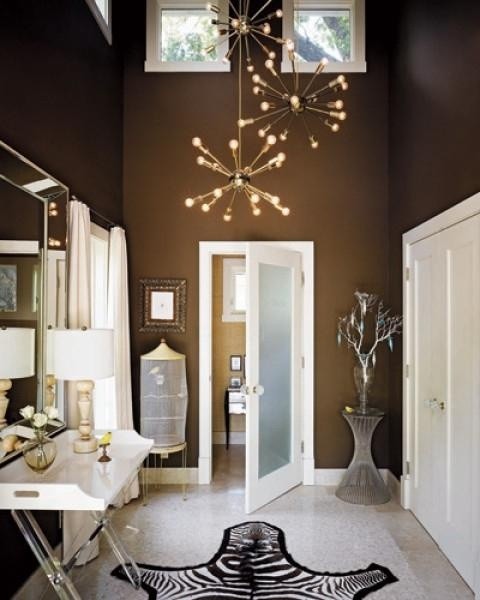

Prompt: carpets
[84,493,429,600]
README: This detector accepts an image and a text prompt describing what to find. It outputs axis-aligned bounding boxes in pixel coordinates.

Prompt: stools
[142,442,188,506]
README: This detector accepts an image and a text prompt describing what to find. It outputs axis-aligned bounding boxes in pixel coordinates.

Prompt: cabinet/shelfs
[402,192,480,600]
[0,429,155,600]
[225,390,247,450]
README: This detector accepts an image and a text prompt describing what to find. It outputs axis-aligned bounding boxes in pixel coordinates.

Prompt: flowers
[19,405,47,472]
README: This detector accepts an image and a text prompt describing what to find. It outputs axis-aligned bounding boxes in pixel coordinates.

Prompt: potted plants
[337,287,403,415]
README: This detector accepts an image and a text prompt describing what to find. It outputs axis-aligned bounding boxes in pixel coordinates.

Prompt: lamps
[54,325,114,453]
[185,0,348,222]
[0,327,36,429]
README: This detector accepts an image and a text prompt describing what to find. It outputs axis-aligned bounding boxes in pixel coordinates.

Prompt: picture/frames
[138,279,186,334]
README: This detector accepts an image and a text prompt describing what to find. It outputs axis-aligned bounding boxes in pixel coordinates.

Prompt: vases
[22,432,57,475]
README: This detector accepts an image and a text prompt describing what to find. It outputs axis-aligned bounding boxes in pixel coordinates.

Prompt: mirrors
[0,140,70,470]
